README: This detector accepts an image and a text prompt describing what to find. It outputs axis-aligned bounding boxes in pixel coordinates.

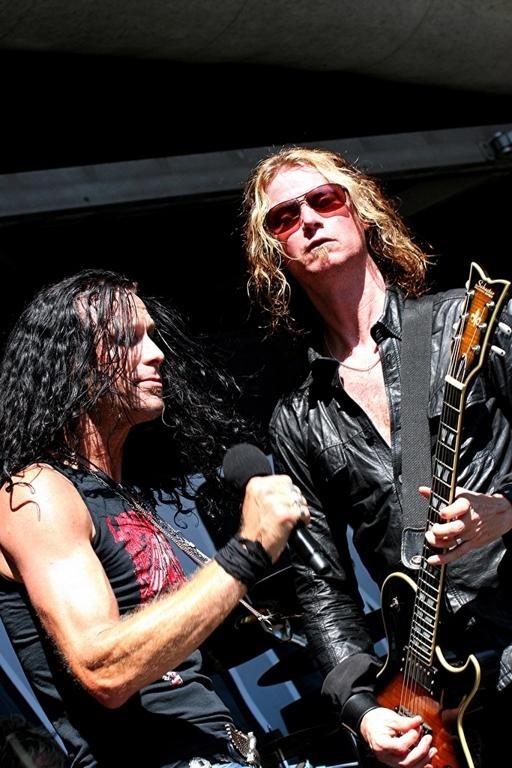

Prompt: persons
[242,149,512,768]
[1,266,313,768]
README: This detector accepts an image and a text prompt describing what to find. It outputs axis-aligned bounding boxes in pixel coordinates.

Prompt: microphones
[220,439,333,576]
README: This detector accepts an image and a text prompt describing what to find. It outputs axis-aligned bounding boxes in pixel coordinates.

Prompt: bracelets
[215,535,274,586]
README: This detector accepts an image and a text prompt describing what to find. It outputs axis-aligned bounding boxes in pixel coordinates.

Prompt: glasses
[261,183,351,236]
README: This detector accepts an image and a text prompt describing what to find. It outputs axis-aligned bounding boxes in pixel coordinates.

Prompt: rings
[455,536,462,546]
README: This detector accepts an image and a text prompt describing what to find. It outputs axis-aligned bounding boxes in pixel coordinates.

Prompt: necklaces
[322,335,380,372]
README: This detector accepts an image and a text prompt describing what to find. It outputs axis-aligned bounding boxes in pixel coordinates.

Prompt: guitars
[358,262,511,768]
